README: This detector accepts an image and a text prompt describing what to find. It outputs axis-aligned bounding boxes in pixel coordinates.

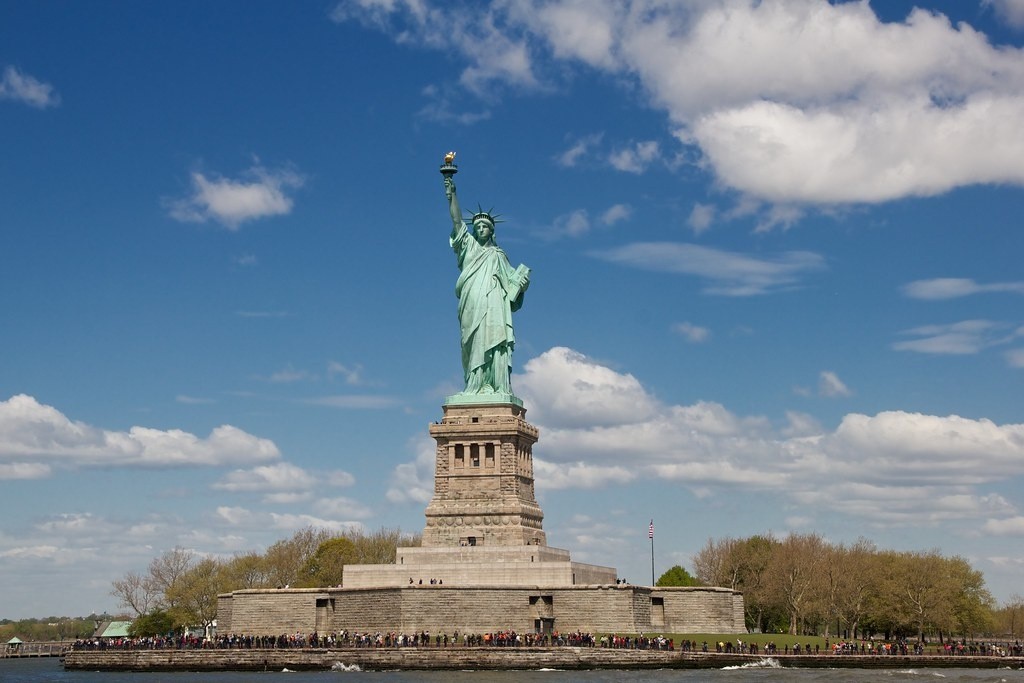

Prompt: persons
[76,629,1023,657]
[444,177,531,396]
